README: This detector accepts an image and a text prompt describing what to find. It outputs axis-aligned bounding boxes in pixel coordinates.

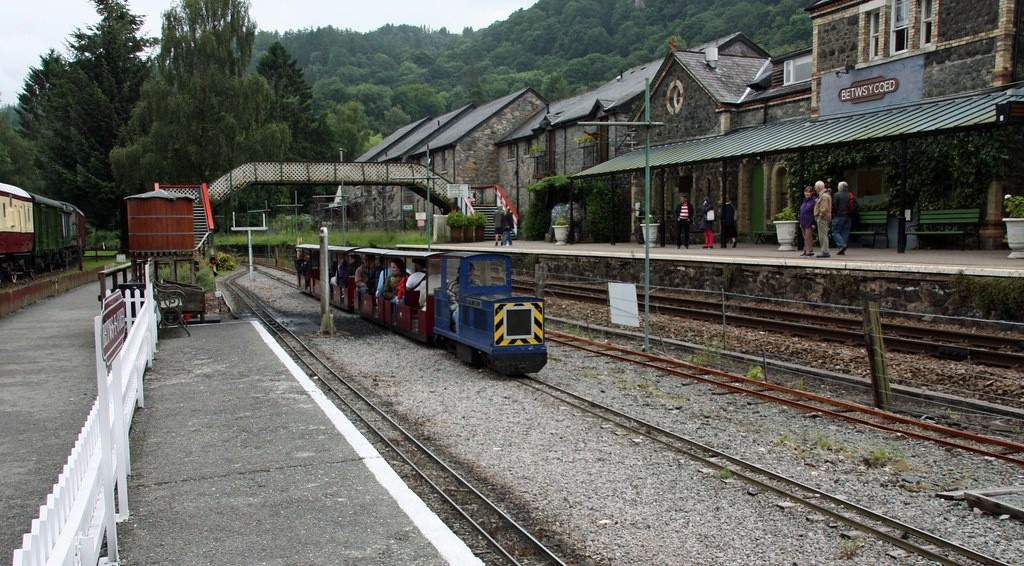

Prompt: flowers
[1003,194,1024,218]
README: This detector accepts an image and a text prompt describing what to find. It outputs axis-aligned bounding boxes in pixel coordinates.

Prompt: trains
[0,183,87,284]
[295,244,547,376]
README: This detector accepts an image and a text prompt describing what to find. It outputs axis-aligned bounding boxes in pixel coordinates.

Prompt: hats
[412,258,428,267]
[362,254,376,260]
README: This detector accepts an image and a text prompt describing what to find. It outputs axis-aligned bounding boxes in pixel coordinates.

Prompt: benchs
[153,279,191,338]
[904,207,981,250]
[849,210,889,249]
[752,219,777,245]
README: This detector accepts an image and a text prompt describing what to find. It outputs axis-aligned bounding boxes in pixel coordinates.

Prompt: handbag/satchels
[848,192,858,216]
[734,209,739,221]
[706,209,715,221]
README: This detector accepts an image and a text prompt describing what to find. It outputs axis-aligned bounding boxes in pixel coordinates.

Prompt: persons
[295,250,427,326]
[799,186,817,256]
[446,263,482,335]
[813,181,832,258]
[493,204,514,247]
[699,198,714,248]
[829,181,851,255]
[724,195,739,248]
[675,195,694,249]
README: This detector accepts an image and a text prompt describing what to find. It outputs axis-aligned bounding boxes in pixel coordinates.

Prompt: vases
[1002,218,1024,259]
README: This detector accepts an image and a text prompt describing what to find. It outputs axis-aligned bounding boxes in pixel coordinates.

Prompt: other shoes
[817,252,831,257]
[801,251,815,257]
[703,245,713,248]
[841,245,847,255]
[733,240,737,248]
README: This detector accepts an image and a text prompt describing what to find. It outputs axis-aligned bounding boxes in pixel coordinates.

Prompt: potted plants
[772,206,799,251]
[445,209,487,243]
[528,143,545,158]
[576,136,595,147]
[640,216,660,247]
[551,217,570,246]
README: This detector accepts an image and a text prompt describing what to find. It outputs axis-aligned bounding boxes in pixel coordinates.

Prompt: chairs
[309,268,450,336]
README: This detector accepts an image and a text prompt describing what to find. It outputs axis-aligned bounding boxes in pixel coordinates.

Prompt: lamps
[836,70,849,78]
